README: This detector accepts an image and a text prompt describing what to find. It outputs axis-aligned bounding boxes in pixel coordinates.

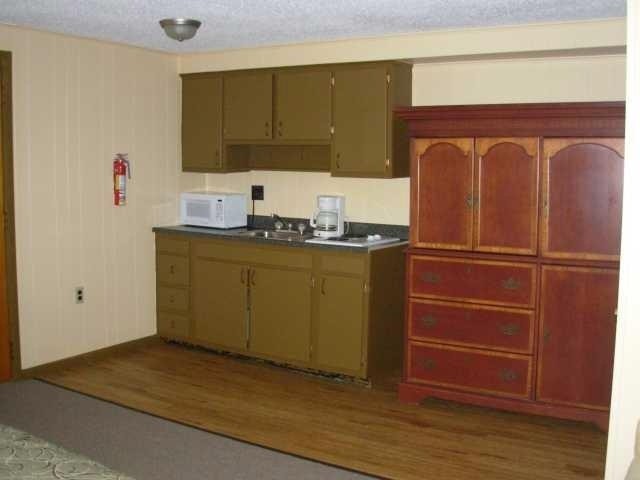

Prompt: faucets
[271,213,292,231]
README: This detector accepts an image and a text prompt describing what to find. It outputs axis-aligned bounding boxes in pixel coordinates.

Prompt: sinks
[238,228,313,247]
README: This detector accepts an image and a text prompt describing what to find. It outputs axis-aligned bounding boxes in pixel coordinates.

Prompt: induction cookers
[305,234,401,247]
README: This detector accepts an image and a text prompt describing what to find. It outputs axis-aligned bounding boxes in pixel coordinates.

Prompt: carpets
[0,422,138,480]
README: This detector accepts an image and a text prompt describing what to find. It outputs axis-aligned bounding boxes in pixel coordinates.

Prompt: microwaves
[179,191,248,229]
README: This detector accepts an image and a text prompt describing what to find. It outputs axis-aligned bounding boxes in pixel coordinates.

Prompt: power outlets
[251,185,264,201]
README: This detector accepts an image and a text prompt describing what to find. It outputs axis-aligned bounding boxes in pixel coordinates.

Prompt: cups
[297,221,308,236]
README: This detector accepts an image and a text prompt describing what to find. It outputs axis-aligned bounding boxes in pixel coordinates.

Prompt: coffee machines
[309,196,346,237]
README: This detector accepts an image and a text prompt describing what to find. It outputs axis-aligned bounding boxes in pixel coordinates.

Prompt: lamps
[159,17,201,42]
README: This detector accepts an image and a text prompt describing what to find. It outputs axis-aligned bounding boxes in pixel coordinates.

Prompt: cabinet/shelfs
[538,261,619,412]
[540,133,625,258]
[405,133,541,256]
[330,67,413,179]
[190,236,314,377]
[224,71,333,145]
[181,77,250,174]
[154,234,190,347]
[397,249,539,403]
[314,243,406,387]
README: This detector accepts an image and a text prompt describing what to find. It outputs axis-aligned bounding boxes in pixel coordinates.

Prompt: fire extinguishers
[113,154,131,206]
